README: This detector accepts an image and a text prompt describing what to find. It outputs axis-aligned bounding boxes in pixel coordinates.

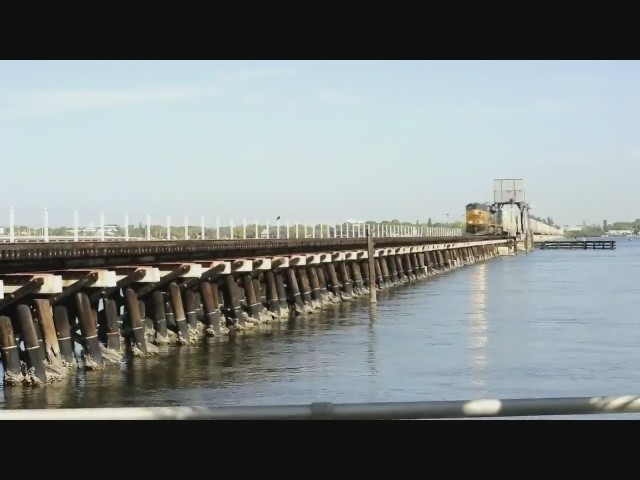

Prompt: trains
[466,202,562,235]
